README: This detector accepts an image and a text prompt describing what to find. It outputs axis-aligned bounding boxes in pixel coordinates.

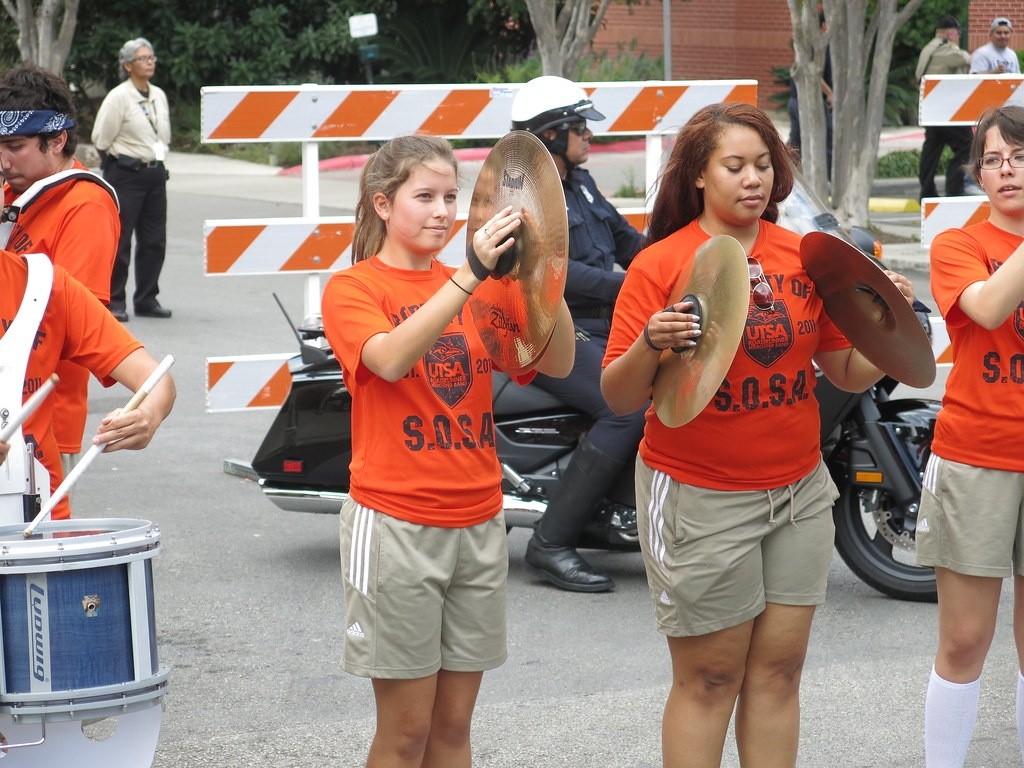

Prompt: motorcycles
[223,148,942,604]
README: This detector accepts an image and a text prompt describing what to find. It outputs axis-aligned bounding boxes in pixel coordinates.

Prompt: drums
[0,515,174,724]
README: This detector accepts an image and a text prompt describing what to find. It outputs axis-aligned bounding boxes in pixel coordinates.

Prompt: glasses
[571,119,587,135]
[127,55,158,64]
[747,255,775,313]
[979,154,1024,170]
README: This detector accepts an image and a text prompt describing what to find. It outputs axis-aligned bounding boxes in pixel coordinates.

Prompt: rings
[484,229,491,238]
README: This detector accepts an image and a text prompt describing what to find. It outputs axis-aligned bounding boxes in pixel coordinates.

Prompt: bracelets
[450,277,473,296]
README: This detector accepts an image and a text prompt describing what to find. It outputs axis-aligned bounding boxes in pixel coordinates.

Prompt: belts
[108,155,163,168]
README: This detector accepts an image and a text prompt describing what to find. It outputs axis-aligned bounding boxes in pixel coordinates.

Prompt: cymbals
[799,230,939,389]
[464,129,572,378]
[651,233,752,430]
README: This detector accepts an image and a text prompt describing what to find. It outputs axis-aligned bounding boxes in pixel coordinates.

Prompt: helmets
[510,76,605,137]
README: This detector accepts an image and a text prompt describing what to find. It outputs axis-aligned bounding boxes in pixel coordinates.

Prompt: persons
[787,10,832,179]
[969,18,1021,74]
[0,249,176,525]
[600,102,914,767]
[914,15,973,205]
[92,38,172,321]
[923,104,1024,768]
[321,138,575,767]
[466,151,542,368]
[0,62,121,454]
[513,76,648,591]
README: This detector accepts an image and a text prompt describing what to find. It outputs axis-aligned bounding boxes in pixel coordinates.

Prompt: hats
[936,16,962,29]
[989,17,1013,36]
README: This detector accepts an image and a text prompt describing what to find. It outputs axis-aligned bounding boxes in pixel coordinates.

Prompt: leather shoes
[135,299,171,316]
[110,309,128,321]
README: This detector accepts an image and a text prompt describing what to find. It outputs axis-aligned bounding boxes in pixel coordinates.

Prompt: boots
[524,435,625,593]
